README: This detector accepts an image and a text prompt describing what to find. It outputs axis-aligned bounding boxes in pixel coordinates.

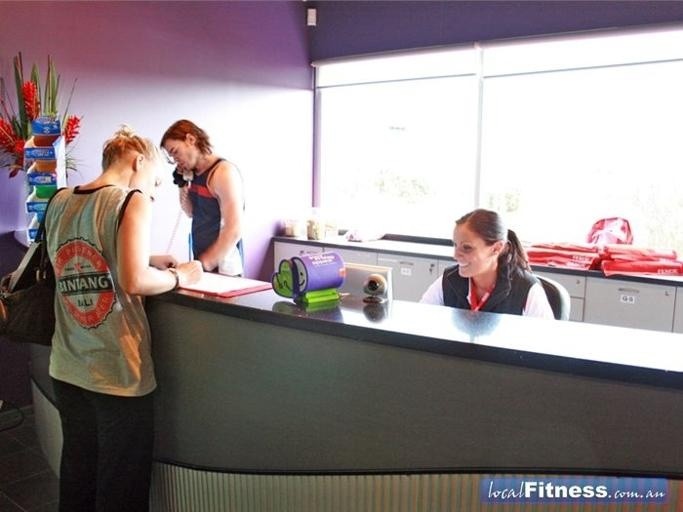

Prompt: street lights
[0,272,56,345]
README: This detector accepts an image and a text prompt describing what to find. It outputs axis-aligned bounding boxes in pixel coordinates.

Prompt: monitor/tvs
[182,270,273,296]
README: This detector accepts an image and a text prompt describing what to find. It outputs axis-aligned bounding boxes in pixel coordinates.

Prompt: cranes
[178,271,273,298]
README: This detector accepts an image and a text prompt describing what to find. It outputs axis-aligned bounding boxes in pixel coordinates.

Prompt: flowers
[0,51,91,186]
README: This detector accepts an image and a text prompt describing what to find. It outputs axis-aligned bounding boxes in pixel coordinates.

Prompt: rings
[189,233,193,262]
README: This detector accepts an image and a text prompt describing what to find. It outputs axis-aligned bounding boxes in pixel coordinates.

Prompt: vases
[0,51,91,186]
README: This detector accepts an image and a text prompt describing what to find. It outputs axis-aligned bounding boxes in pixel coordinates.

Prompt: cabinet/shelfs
[272,234,683,333]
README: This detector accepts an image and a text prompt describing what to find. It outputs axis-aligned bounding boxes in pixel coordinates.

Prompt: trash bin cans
[166,267,182,286]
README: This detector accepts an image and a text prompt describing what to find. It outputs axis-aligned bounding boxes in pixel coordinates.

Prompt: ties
[337,263,393,300]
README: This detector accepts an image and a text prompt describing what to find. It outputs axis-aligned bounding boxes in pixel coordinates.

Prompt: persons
[159,119,246,276]
[422,208,557,320]
[45,131,204,512]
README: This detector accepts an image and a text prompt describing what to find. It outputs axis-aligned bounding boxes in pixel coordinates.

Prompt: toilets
[183,168,194,182]
